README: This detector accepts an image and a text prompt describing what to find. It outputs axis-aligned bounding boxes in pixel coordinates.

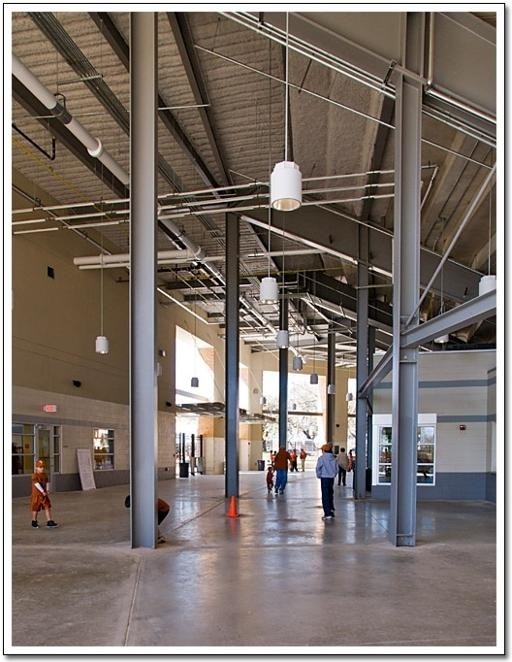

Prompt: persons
[380,446,432,463]
[93,446,108,466]
[30,460,60,529]
[124,494,171,544]
[266,443,353,521]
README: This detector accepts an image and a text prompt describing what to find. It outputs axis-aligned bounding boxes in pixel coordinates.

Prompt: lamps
[258,347,297,410]
[261,209,278,305]
[277,215,354,401]
[94,159,109,355]
[269,11,302,212]
[190,290,200,388]
[433,176,496,344]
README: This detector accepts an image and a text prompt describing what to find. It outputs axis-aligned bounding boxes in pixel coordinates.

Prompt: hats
[36,459,46,467]
[320,444,331,451]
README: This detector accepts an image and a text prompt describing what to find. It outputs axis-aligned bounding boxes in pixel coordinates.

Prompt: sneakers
[32,520,38,528]
[322,509,335,520]
[47,520,59,527]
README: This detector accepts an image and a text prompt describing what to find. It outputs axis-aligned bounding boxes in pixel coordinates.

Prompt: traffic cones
[225,494,241,518]
